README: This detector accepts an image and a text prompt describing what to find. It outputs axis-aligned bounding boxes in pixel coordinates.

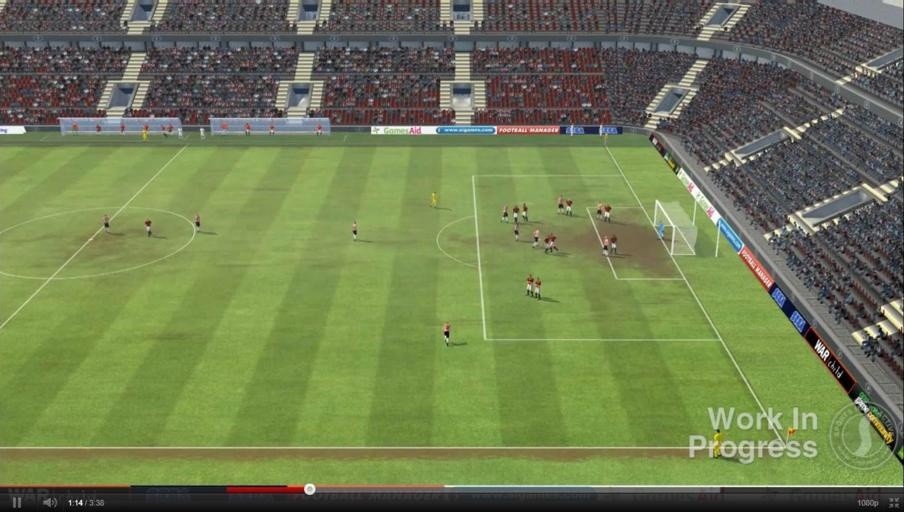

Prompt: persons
[429,191,438,208]
[104,214,110,232]
[195,213,201,232]
[441,321,450,346]
[352,220,358,240]
[144,217,152,238]
[657,220,665,241]
[713,429,722,458]
[501,194,622,300]
